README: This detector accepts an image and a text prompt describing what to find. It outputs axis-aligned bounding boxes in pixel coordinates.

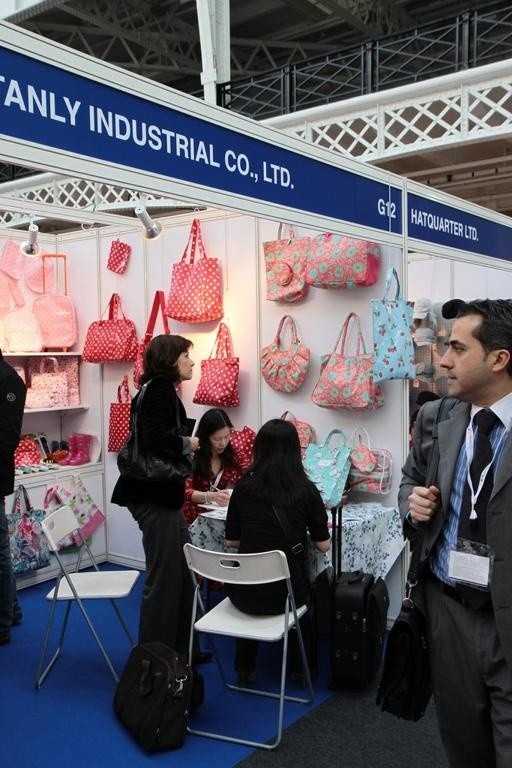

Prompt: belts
[426,565,460,602]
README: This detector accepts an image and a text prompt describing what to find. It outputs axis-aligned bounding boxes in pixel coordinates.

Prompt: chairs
[180,540,315,754]
[33,505,141,689]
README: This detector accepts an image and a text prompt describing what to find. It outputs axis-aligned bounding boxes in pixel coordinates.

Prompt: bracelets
[205,491,210,505]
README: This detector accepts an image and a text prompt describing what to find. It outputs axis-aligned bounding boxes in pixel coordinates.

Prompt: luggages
[33,253,79,352]
[304,567,388,698]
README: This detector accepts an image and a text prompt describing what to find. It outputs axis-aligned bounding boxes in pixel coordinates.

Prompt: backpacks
[111,635,195,757]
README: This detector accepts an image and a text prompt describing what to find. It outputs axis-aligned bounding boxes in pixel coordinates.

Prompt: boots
[59,433,93,467]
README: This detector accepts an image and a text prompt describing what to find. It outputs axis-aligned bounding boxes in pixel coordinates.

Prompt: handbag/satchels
[4,478,108,576]
[6,356,81,409]
[377,580,434,724]
[260,313,309,395]
[303,231,381,291]
[367,264,418,384]
[193,323,243,407]
[133,290,182,392]
[261,220,312,304]
[107,376,132,453]
[163,215,224,326]
[107,239,132,273]
[276,410,395,511]
[311,311,386,412]
[83,293,139,363]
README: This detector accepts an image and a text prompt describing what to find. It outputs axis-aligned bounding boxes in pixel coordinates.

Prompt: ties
[453,408,505,615]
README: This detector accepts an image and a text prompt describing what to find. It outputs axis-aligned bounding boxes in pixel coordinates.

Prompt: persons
[398,298,512,768]
[181,408,243,592]
[111,334,213,665]
[0,349,27,646]
[223,419,332,689]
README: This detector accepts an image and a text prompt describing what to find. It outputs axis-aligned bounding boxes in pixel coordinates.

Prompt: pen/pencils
[211,485,225,495]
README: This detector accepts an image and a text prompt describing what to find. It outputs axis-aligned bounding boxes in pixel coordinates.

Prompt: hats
[413,296,468,407]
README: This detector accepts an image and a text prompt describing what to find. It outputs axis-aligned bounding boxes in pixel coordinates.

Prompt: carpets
[2,559,340,767]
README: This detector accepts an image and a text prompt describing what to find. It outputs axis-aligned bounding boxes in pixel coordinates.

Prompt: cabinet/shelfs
[1,348,109,592]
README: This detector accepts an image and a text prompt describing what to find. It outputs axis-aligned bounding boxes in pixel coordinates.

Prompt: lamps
[134,191,164,242]
[20,212,40,257]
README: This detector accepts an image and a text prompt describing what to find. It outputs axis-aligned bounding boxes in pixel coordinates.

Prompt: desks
[190,499,409,673]
